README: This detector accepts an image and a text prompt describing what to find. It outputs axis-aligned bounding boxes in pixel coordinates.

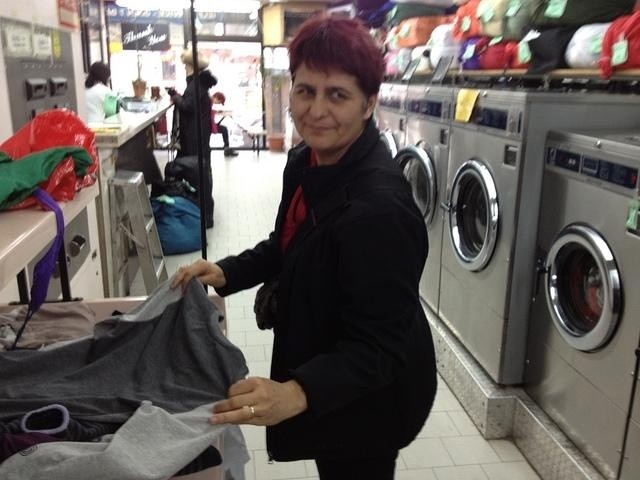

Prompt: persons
[170,15,437,479]
[77,61,120,126]
[210,91,239,156]
[165,48,217,229]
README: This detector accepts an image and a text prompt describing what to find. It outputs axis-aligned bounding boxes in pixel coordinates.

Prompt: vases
[134,81,146,98]
[268,137,284,150]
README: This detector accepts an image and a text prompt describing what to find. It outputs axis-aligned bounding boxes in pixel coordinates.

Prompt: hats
[181,46,209,69]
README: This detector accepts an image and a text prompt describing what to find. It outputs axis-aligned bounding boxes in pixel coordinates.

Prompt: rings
[251,406,255,416]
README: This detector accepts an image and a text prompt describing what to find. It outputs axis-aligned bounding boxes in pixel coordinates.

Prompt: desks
[0,177,106,304]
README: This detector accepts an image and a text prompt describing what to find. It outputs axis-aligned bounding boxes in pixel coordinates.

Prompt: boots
[224,148,239,156]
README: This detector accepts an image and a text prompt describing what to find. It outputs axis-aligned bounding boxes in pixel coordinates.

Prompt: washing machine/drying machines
[524,126,640,479]
[442,87,638,390]
[372,80,453,325]
